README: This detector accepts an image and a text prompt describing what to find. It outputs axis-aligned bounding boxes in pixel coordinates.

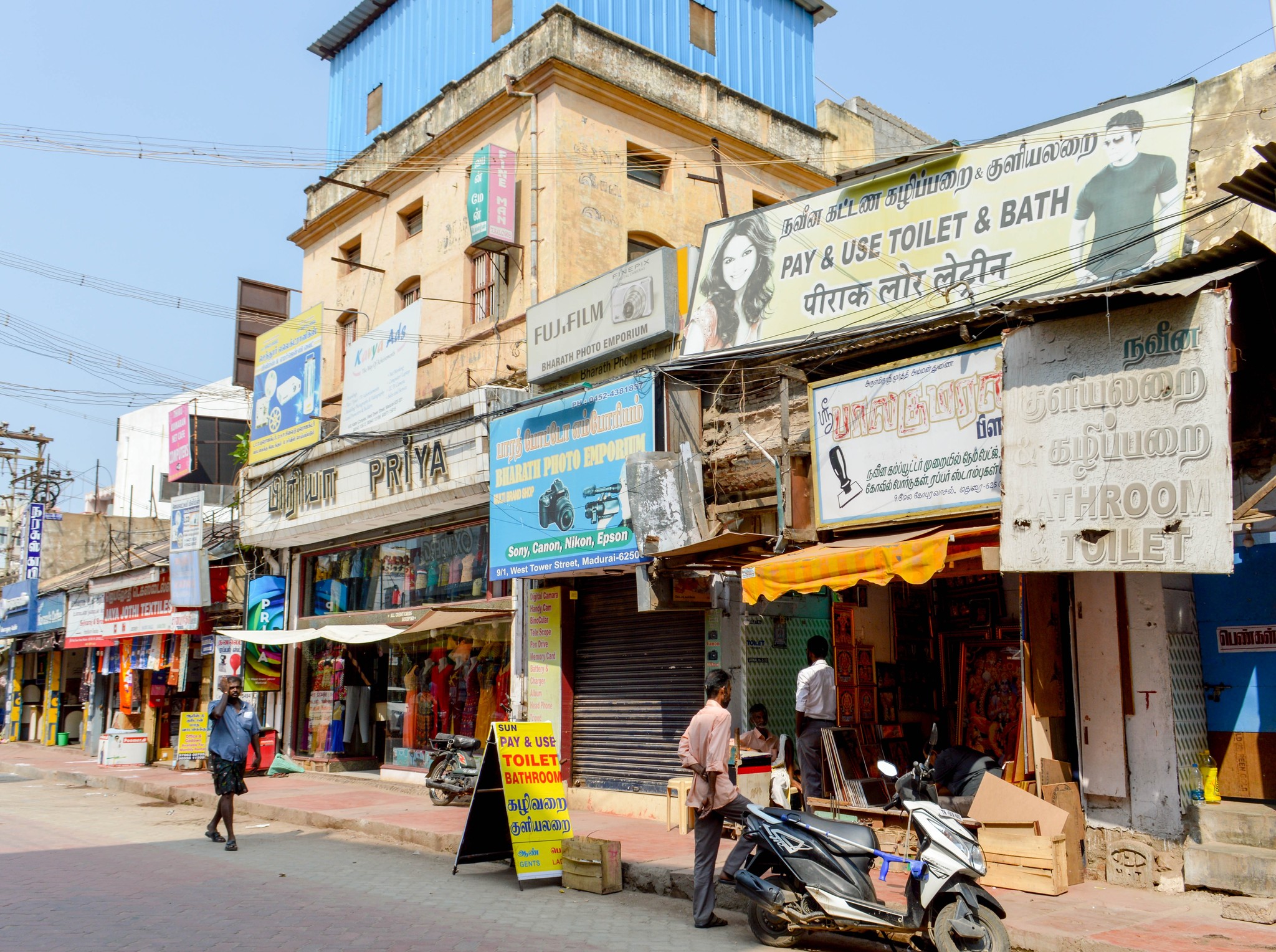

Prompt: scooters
[734,722,1012,951]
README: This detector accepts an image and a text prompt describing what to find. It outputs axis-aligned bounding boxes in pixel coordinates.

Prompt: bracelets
[224,691,229,695]
[748,747,751,751]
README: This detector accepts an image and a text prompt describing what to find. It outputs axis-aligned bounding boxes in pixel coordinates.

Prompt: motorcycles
[425,694,515,806]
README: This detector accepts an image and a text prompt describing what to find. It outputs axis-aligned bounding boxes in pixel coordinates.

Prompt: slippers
[205,832,226,842]
[694,914,728,928]
[225,841,237,850]
[719,877,737,885]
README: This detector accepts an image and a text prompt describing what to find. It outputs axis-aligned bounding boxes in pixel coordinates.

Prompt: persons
[206,676,263,851]
[677,215,779,354]
[306,547,511,752]
[171,510,183,551]
[677,636,838,928]
[1070,110,1184,283]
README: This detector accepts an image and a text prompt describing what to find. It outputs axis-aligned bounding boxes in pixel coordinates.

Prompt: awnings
[742,523,1001,608]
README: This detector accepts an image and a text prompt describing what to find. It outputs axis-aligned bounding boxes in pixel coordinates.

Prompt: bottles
[1189,763,1206,805]
[1198,750,1222,804]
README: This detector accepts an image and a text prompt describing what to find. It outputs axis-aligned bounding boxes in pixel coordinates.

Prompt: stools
[666,777,698,835]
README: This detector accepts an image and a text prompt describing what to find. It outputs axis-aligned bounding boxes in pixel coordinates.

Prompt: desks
[97,733,147,769]
[806,799,979,828]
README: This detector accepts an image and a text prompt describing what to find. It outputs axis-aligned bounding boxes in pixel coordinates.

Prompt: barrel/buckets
[57,732,70,746]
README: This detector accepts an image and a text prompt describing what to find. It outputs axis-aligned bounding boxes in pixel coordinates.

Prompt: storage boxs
[561,835,623,894]
[978,829,1070,898]
[112,711,142,729]
